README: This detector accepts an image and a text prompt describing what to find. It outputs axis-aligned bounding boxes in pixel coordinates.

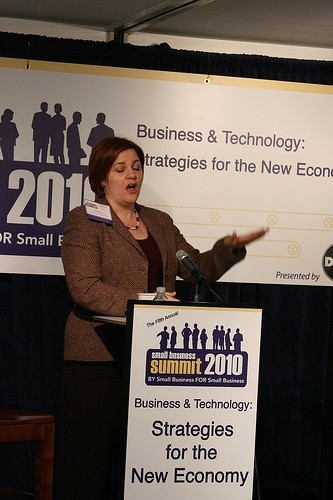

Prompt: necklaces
[126,207,139,229]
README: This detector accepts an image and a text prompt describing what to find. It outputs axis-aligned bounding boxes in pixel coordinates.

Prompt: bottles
[153,286,168,301]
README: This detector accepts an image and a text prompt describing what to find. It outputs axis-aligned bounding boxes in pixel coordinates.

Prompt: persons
[59,134,272,499]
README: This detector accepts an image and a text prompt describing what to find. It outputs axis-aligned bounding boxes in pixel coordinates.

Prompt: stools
[0,411,56,500]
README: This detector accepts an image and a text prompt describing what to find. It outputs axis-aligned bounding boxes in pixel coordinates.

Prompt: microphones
[176,250,209,287]
